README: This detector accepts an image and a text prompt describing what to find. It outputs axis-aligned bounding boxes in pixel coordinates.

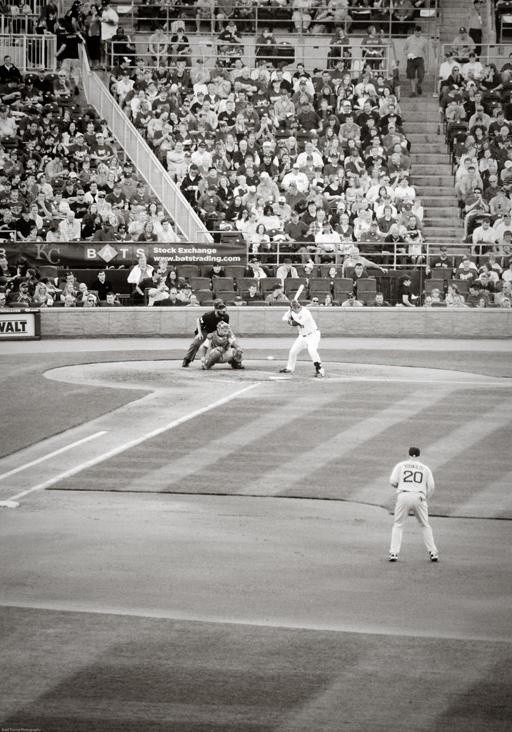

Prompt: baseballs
[267,356,274,361]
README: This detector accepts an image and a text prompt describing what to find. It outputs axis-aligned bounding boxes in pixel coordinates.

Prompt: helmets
[214,321,232,336]
[212,298,227,310]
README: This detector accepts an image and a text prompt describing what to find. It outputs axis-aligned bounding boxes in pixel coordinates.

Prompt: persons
[279,300,325,377]
[199,321,244,371]
[182,298,229,366]
[390,447,439,562]
[0,0,512,308]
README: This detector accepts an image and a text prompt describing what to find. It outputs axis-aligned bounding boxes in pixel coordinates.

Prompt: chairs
[0,0,512,309]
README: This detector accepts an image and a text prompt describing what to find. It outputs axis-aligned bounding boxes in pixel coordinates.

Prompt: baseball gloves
[233,348,244,362]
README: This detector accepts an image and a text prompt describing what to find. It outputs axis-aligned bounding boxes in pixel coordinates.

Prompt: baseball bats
[286,284,304,322]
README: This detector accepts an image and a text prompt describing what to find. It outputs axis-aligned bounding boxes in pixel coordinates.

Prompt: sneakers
[278,366,324,378]
[429,552,438,560]
[179,360,244,372]
[388,552,398,562]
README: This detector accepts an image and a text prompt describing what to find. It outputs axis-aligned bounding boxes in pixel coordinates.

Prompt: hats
[290,299,301,309]
[407,447,421,459]
